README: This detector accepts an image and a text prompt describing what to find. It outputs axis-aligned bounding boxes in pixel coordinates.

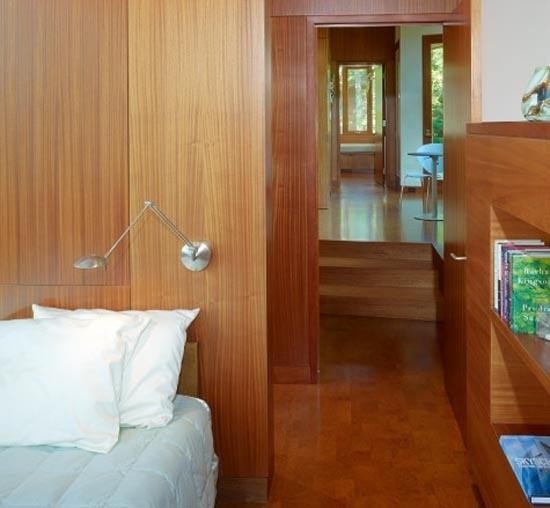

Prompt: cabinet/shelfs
[467,121,550,507]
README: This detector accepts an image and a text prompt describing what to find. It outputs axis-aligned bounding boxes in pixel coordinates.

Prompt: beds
[1,342,219,508]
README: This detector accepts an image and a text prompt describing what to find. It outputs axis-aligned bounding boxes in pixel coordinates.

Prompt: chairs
[399,143,444,208]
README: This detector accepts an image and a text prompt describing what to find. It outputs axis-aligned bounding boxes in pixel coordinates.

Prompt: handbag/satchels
[521,66,550,121]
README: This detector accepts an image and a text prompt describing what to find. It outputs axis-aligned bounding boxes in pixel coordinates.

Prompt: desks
[398,152,445,222]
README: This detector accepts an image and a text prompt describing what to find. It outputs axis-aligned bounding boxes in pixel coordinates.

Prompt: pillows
[31,303,200,429]
[0,313,151,455]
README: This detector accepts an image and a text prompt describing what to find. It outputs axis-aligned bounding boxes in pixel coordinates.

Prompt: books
[493,239,550,341]
[498,435,550,505]
